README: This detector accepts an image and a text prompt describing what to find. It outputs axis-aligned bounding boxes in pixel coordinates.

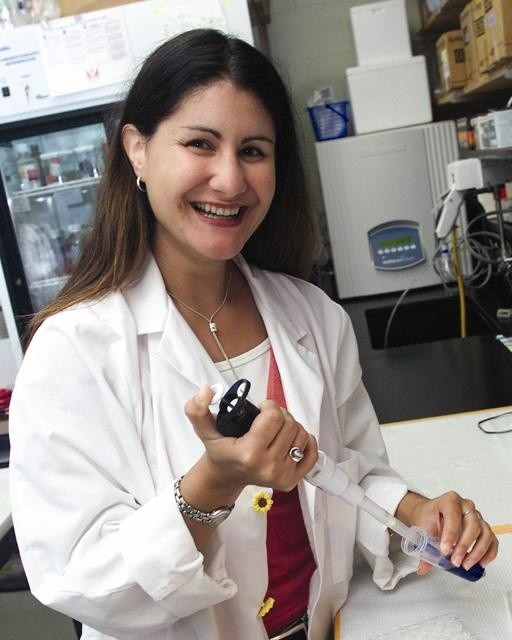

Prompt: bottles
[399,525,485,582]
[61,224,92,275]
[13,139,100,193]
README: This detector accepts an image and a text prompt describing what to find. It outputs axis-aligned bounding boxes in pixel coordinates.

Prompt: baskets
[306,102,348,141]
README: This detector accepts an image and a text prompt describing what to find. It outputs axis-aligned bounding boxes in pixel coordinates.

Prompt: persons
[6,27,501,639]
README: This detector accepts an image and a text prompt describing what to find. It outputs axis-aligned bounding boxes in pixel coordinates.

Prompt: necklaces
[164,262,252,392]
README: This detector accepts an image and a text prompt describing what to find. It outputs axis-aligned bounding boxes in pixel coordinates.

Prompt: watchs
[173,476,235,527]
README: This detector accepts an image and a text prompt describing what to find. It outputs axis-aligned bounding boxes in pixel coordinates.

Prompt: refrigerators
[0,1,271,376]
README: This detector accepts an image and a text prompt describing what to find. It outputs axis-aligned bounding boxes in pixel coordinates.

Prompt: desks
[329,403,512,639]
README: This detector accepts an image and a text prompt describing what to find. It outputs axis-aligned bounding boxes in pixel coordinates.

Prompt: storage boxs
[347,0,432,137]
[434,0,512,149]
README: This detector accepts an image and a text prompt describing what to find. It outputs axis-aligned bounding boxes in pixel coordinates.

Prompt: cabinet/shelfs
[412,0,512,159]
[0,97,126,361]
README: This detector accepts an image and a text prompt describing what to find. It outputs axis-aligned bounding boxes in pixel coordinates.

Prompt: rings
[288,447,304,463]
[463,509,477,517]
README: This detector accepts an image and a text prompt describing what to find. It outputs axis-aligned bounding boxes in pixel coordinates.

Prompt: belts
[266,626,308,640]
